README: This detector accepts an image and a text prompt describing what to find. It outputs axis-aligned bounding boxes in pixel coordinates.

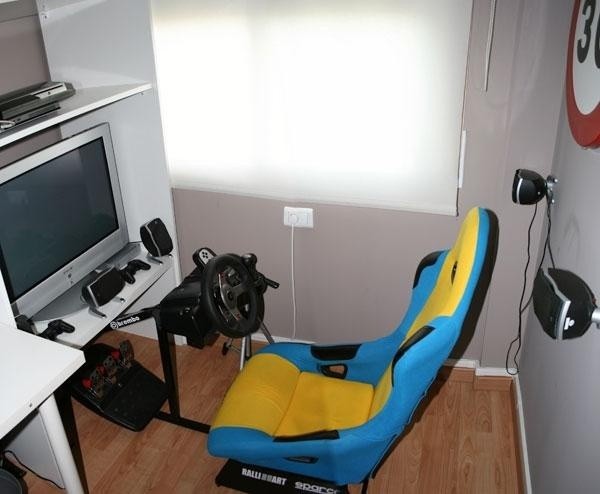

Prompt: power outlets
[284,206,314,228]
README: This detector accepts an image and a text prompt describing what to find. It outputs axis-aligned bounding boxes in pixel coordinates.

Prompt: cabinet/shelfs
[0,84,173,355]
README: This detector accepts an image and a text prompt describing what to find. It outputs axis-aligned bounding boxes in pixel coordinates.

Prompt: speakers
[140,218,173,264]
[533,267,596,340]
[80,267,125,317]
[512,168,547,205]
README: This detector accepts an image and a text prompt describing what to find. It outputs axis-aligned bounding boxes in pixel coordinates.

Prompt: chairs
[206,206,498,494]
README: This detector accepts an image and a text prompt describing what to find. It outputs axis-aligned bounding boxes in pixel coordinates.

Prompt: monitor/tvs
[0,120,129,322]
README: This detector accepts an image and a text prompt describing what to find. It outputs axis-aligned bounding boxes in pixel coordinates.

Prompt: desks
[1,326,88,494]
